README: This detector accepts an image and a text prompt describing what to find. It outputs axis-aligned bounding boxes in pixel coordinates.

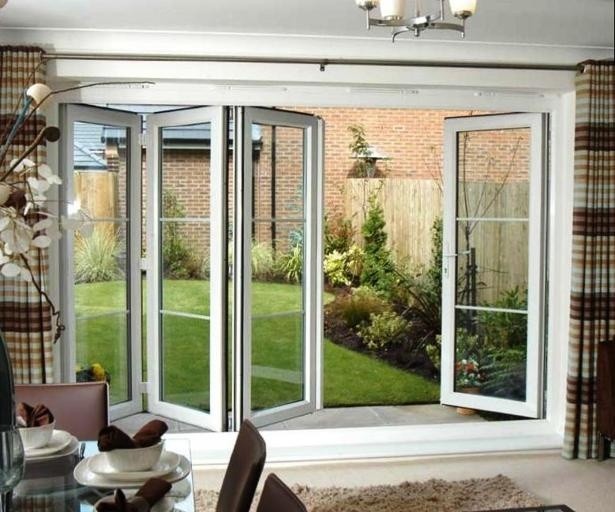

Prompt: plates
[86,449,181,482]
[72,451,193,489]
[21,429,81,465]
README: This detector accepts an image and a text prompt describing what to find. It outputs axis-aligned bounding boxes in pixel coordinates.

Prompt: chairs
[14,382,107,440]
[214,418,307,511]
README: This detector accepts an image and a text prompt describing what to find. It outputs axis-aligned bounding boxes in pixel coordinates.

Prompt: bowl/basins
[15,416,56,450]
[107,437,164,471]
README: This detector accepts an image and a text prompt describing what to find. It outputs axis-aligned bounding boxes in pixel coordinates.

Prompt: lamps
[355,0,476,41]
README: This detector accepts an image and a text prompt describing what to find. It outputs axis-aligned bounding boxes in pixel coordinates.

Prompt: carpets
[195,474,572,509]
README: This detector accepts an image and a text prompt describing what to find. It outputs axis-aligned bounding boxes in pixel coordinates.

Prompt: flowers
[0,57,153,341]
